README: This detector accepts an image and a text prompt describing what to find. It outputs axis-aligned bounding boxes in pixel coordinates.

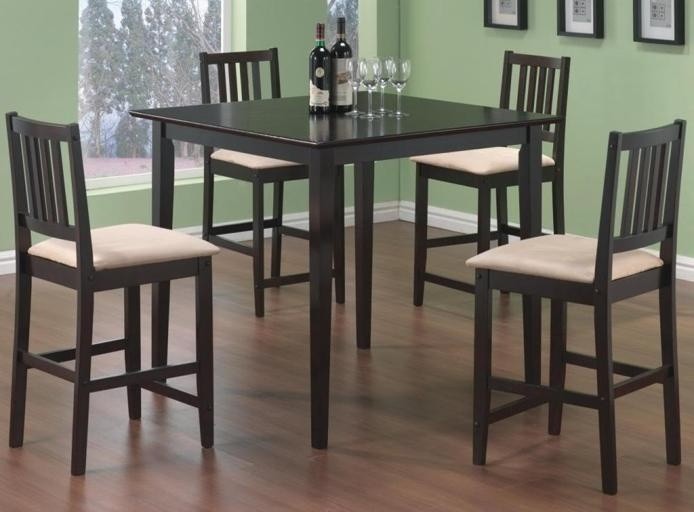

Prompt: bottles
[331,17,354,114]
[308,22,331,115]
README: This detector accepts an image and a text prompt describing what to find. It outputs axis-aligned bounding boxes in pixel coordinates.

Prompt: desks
[127,92,564,450]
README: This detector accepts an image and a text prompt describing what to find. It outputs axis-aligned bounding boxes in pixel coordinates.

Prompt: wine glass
[344,57,411,120]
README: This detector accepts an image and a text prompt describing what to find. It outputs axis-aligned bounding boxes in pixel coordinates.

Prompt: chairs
[411,49,571,306]
[5,110,220,476]
[198,47,347,317]
[465,117,689,497]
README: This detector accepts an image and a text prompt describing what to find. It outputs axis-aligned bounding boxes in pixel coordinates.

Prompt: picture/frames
[483,0,687,46]
